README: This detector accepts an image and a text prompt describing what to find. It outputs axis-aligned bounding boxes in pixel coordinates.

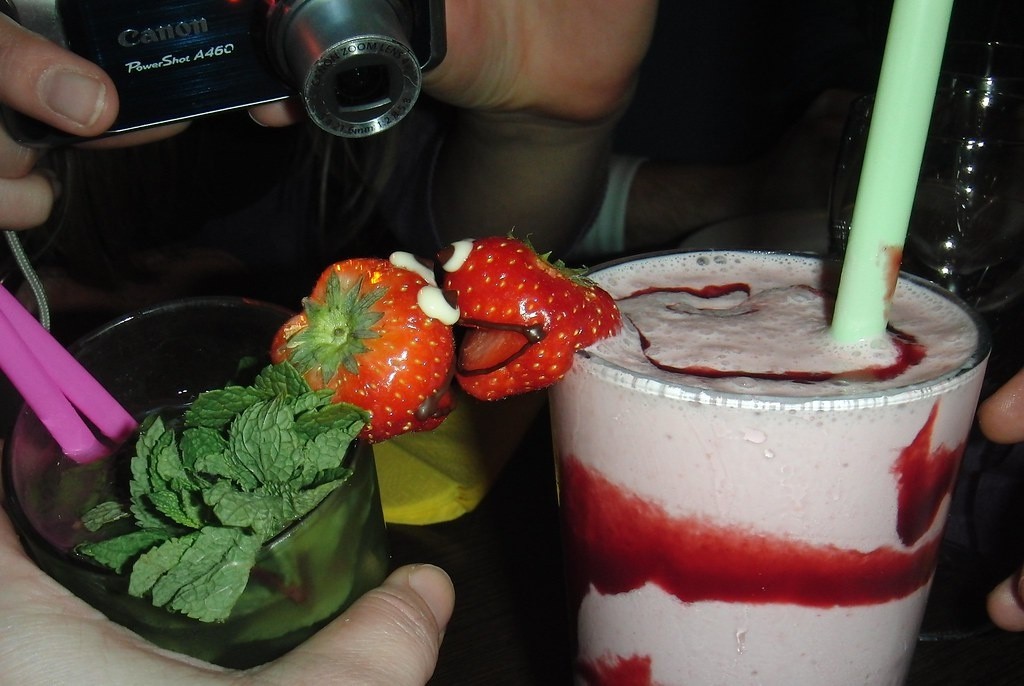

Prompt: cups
[829,39,1023,311]
[547,244,995,686]
[6,298,390,670]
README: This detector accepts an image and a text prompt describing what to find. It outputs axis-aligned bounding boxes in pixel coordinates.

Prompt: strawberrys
[432,237,623,401]
[272,258,455,446]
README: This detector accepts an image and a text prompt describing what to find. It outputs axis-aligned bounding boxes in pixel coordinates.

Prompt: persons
[1,0,1022,686]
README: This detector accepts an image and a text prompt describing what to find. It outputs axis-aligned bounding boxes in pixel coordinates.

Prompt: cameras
[1,0,448,152]
